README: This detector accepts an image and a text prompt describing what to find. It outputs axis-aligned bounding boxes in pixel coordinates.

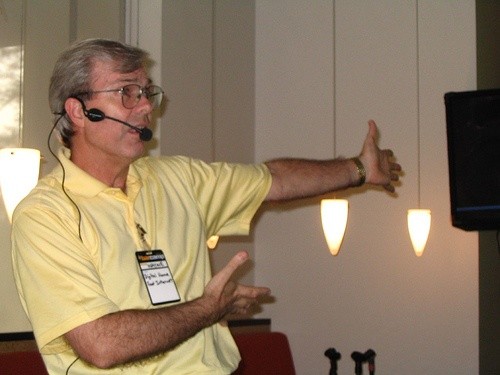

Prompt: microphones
[105,115,152,142]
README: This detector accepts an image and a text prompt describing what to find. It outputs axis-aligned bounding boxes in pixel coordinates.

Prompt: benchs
[0,316,296,375]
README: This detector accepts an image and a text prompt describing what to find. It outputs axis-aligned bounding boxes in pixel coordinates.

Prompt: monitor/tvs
[444,89,500,232]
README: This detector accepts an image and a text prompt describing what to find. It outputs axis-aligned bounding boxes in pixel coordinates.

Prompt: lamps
[0,0,40,225]
[319,0,349,257]
[407,0,432,256]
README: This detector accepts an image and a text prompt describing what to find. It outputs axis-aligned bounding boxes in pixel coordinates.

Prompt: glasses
[70,84,166,110]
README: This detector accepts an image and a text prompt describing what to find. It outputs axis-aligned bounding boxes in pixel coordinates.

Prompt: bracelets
[351,158,367,186]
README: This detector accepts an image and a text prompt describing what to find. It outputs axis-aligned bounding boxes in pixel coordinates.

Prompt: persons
[10,38,401,375]
